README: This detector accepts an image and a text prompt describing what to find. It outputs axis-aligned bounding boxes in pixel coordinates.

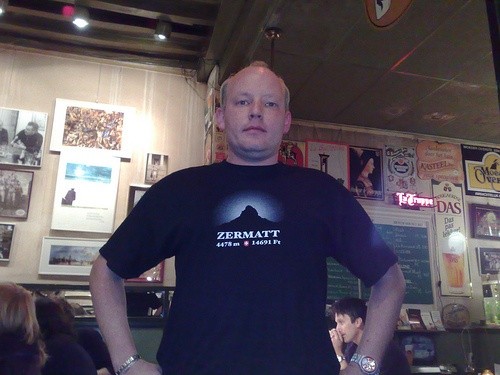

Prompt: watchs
[351,354,380,375]
[337,356,345,362]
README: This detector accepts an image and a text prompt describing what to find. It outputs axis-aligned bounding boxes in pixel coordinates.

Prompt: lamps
[154,21,172,41]
[72,6,89,27]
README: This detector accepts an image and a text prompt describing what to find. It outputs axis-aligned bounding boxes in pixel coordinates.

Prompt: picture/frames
[471,203,500,241]
[50,148,121,233]
[0,167,34,220]
[0,107,48,167]
[0,223,16,261]
[305,139,385,201]
[39,236,109,276]
[49,98,134,160]
[128,186,149,217]
[126,260,165,282]
[478,246,500,275]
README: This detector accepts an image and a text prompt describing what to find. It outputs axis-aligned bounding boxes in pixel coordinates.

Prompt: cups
[439,362,454,375]
[441,251,465,294]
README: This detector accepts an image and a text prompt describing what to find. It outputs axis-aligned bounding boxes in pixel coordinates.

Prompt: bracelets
[115,354,143,375]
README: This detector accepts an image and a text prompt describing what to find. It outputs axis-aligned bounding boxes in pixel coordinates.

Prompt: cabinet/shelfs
[394,326,500,375]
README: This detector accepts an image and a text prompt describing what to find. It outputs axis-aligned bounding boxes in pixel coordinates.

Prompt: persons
[328,297,410,375]
[0,282,47,375]
[35,297,96,375]
[90,65,405,375]
[12,122,43,164]
